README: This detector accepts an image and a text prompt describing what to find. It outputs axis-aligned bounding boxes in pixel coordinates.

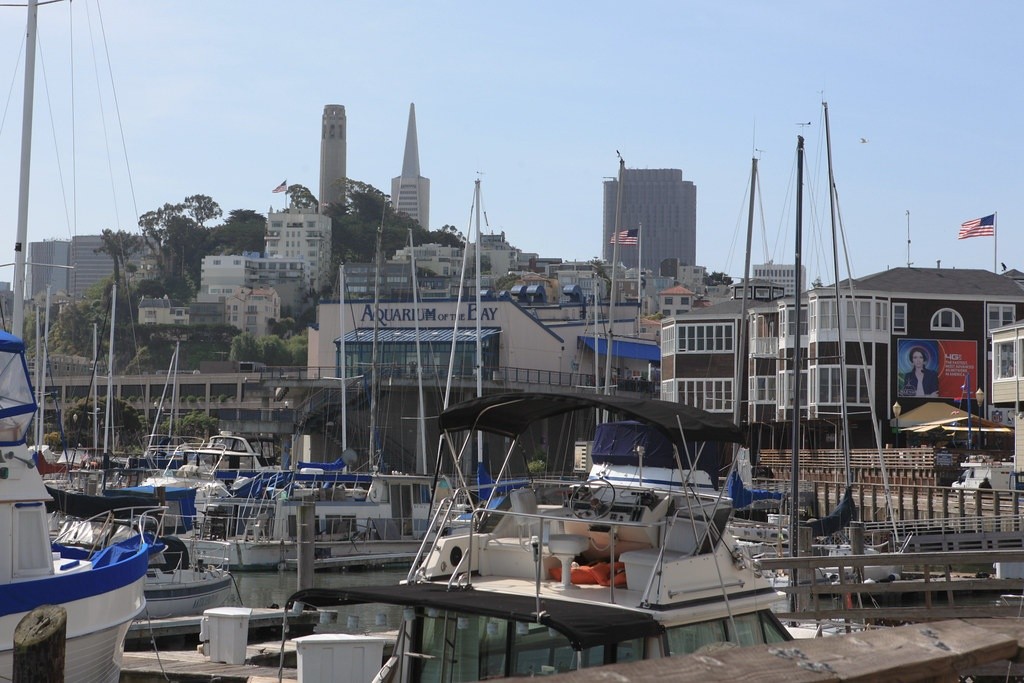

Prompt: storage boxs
[290,632,385,683]
[197,607,251,667]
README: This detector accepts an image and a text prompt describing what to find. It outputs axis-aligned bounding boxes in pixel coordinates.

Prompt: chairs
[509,488,590,593]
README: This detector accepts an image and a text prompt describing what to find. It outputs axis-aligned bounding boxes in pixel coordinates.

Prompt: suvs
[950,460,1016,500]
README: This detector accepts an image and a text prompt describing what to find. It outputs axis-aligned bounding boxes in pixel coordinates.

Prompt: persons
[900,348,939,396]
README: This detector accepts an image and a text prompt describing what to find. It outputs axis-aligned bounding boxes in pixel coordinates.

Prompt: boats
[1,101,896,683]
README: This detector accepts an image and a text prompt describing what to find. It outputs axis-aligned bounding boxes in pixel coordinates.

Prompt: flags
[958,213,994,240]
[609,229,638,246]
[272,180,287,193]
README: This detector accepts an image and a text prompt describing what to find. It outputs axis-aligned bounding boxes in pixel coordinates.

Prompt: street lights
[892,400,901,448]
[976,387,985,453]
